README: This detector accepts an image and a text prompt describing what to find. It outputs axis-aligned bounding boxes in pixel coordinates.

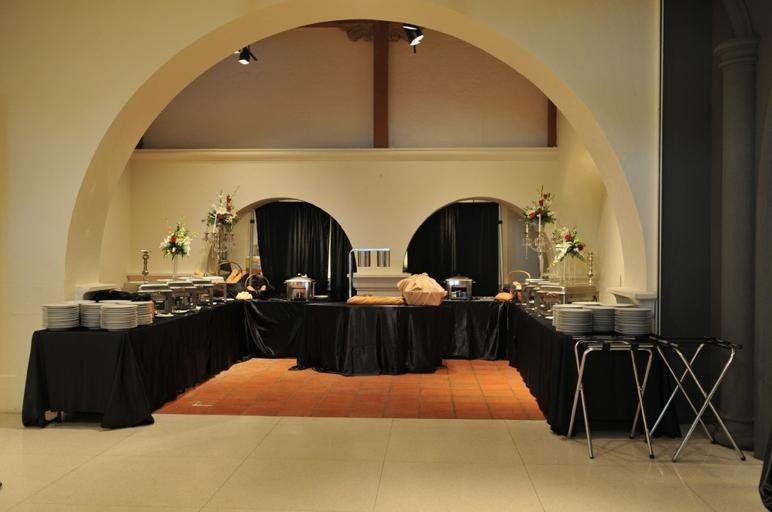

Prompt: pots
[284,273,317,302]
[443,273,477,300]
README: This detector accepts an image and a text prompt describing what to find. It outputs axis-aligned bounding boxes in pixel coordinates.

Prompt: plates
[614,308,652,335]
[606,303,634,307]
[136,299,155,326]
[584,307,614,333]
[80,303,103,329]
[42,302,80,330]
[556,308,592,334]
[571,301,600,306]
[100,303,139,333]
[552,304,583,327]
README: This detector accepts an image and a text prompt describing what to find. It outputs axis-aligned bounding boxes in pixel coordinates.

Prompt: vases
[172,255,179,279]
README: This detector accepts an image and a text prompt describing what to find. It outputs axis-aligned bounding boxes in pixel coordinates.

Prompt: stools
[566,334,658,460]
[649,334,750,464]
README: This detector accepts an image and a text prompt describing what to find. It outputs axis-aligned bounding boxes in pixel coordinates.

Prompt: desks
[507,302,682,438]
[288,300,447,378]
[244,292,329,360]
[441,295,508,361]
[19,297,246,431]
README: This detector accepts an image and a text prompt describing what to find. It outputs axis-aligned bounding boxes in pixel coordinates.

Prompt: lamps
[414,23,425,44]
[236,44,258,67]
[406,27,421,48]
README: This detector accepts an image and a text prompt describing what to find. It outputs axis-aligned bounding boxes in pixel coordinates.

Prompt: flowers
[157,214,201,259]
[520,184,558,225]
[206,190,242,232]
[547,224,587,270]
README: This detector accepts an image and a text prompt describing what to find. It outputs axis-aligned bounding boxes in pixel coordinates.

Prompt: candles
[537,212,542,233]
[525,222,530,237]
